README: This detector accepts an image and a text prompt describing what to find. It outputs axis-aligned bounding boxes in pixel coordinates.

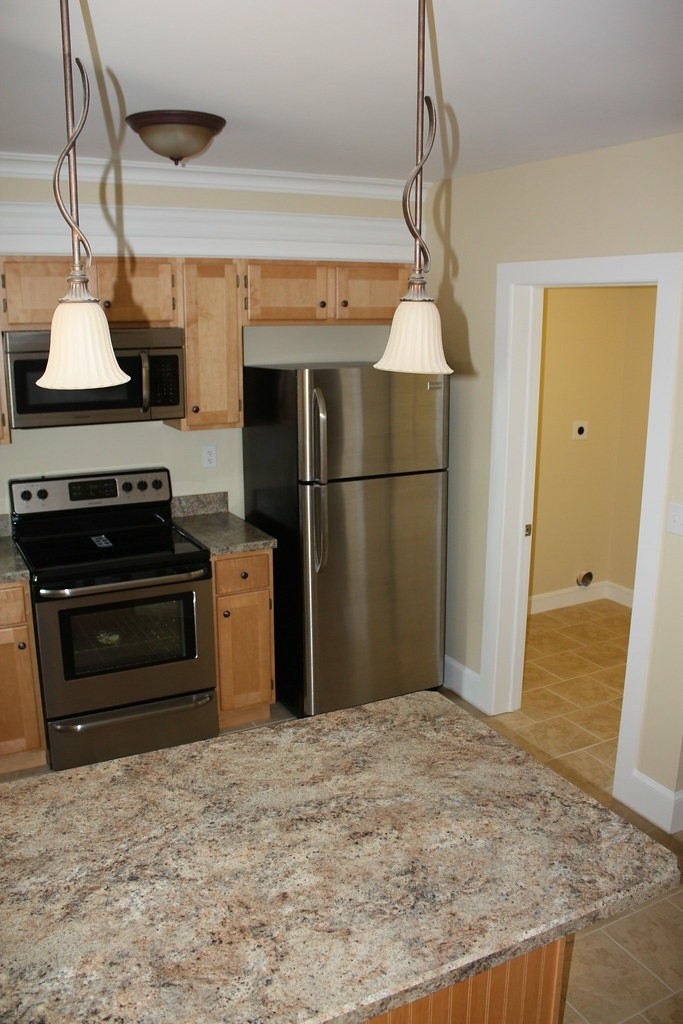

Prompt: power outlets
[202,446,216,469]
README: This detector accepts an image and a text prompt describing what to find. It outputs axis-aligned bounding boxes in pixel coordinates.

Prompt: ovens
[7,466,220,773]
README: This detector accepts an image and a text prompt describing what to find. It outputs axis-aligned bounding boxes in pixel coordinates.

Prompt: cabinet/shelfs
[0,580,49,777]
[243,257,414,326]
[0,254,180,330]
[172,258,247,431]
[211,549,277,731]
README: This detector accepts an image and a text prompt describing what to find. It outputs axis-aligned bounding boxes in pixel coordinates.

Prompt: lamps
[125,109,226,167]
[369,0,455,376]
[30,2,136,391]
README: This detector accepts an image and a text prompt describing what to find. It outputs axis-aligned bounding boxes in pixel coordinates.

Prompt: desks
[1,690,680,1024]
